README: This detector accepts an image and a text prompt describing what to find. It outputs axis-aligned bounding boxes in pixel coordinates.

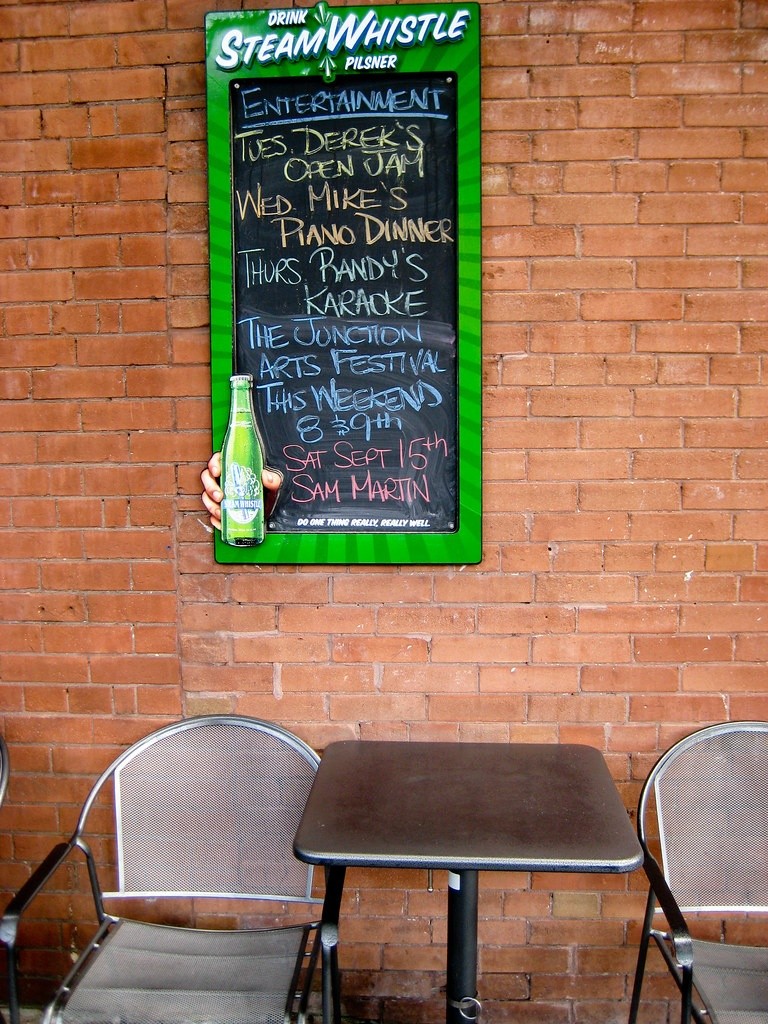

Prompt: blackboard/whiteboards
[204,5,482,562]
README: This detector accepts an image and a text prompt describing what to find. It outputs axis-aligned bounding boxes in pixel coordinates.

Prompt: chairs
[0,711,345,1024]
[627,720,768,1024]
[0,736,10,809]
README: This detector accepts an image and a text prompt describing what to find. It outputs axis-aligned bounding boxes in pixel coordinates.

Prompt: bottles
[218,374,268,548]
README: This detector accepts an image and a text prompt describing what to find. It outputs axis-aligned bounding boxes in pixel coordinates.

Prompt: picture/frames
[201,0,482,564]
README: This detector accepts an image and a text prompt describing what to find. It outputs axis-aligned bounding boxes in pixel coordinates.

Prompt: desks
[290,738,645,1024]
